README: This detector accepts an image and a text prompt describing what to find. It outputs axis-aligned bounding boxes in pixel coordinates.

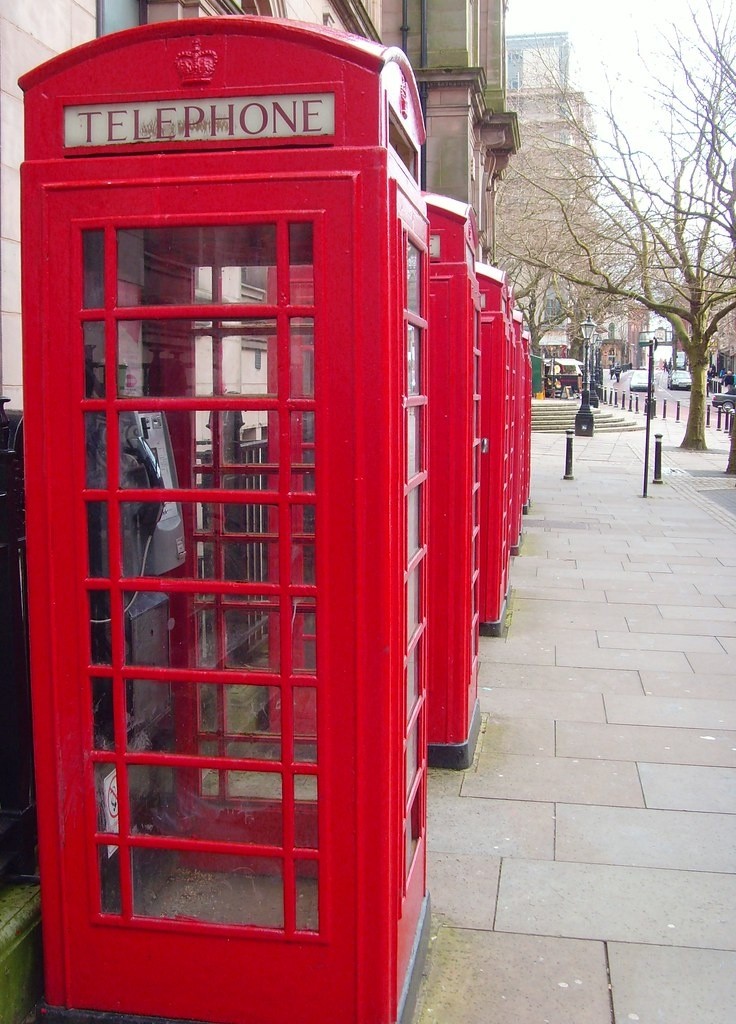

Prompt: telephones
[94,411,187,580]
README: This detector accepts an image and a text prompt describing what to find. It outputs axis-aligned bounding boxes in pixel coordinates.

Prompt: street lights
[575,311,597,437]
[589,331,600,409]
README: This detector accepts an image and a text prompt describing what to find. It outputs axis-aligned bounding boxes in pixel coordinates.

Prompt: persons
[719,368,726,378]
[615,363,622,382]
[724,371,736,386]
[712,364,716,378]
[663,359,667,372]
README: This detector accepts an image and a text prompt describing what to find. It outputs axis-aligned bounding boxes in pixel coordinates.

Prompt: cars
[667,370,691,390]
[629,371,654,392]
[711,388,736,413]
